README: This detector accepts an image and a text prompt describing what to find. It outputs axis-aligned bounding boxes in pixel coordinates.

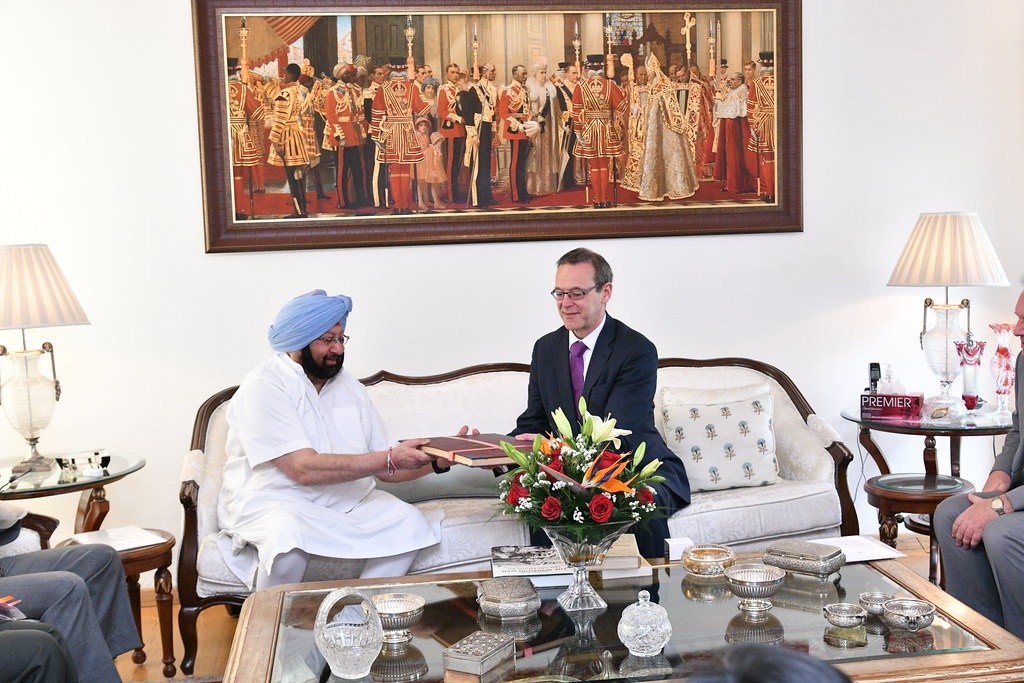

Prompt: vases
[988,323,1016,419]
[545,601,619,681]
[540,520,641,612]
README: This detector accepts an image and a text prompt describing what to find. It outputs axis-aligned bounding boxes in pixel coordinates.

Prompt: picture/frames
[191,0,802,254]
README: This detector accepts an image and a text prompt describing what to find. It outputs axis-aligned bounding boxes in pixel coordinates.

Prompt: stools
[56,528,176,678]
[863,473,975,550]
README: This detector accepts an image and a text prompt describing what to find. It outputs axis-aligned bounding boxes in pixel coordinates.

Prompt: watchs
[992,497,1005,515]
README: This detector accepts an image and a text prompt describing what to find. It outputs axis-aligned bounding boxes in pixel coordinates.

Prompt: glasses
[317,334,350,345]
[550,284,601,300]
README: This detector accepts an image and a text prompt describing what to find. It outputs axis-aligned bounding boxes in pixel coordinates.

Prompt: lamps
[885,212,1011,422]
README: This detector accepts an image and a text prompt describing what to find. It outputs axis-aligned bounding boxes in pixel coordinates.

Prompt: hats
[269,289,351,353]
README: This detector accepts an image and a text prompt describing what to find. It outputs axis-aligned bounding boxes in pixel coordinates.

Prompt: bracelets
[388,447,397,476]
[432,460,450,474]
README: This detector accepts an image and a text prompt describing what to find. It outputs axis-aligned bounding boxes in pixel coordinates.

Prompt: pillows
[660,381,780,492]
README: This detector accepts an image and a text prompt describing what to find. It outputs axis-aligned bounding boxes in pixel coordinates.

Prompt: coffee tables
[222,550,1024,683]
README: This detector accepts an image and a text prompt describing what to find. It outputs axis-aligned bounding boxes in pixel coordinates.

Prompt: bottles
[617,589,672,656]
[619,652,673,679]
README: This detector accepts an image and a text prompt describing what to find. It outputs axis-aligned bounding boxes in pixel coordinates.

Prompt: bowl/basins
[681,544,736,576]
[370,639,428,683]
[680,573,734,603]
[724,564,786,612]
[823,593,936,629]
[367,592,426,643]
[822,616,935,656]
[723,602,785,650]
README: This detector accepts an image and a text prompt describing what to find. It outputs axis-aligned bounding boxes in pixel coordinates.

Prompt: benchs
[177,357,859,677]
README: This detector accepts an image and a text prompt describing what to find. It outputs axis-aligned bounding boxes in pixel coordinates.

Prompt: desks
[0,451,146,535]
[839,407,1014,592]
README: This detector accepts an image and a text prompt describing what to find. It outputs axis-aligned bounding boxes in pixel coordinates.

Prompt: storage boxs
[443,631,516,676]
[444,655,515,683]
[860,391,924,422]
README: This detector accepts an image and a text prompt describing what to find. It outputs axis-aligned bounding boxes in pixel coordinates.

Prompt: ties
[570,343,589,420]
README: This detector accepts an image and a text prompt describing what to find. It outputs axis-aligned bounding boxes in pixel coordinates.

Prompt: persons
[935,289,1024,640]
[218,289,481,591]
[482,248,692,557]
[688,644,852,683]
[1,503,141,682]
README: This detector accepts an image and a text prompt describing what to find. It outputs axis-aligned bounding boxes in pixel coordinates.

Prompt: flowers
[474,395,681,544]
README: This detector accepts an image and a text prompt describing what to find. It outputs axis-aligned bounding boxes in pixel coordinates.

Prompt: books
[425,596,574,657]
[539,576,653,603]
[490,533,652,587]
[397,433,534,467]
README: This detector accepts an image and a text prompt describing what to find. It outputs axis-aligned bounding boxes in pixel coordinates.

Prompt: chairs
[0,512,60,559]
